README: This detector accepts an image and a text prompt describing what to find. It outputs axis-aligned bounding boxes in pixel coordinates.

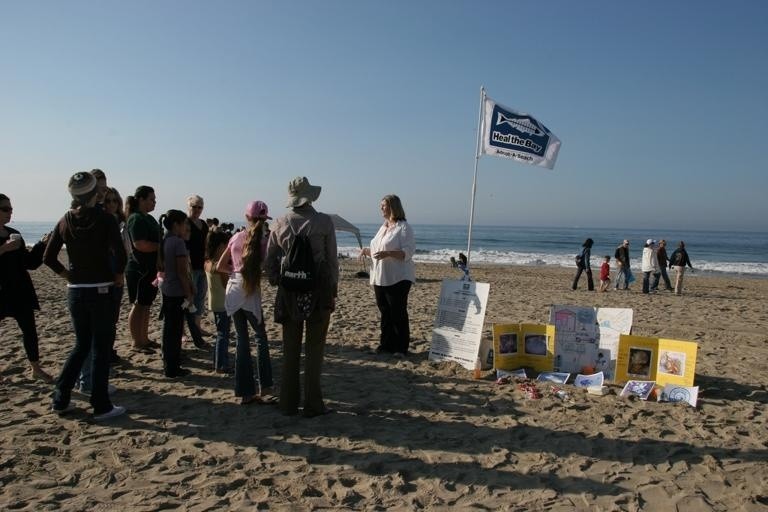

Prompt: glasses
[1,206,13,213]
[106,198,118,203]
[192,205,202,209]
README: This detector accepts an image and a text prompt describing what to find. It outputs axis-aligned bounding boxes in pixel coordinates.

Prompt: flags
[479,96,562,171]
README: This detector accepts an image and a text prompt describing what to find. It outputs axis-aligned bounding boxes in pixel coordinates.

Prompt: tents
[323,213,366,273]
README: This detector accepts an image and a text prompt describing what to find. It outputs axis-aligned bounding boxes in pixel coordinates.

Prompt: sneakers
[94,404,126,420]
[54,401,77,414]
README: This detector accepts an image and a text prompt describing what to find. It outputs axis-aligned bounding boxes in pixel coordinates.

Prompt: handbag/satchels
[126,221,159,265]
[576,248,588,269]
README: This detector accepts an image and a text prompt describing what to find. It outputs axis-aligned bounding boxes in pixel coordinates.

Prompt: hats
[646,238,656,245]
[285,176,322,209]
[68,171,97,198]
[246,199,273,219]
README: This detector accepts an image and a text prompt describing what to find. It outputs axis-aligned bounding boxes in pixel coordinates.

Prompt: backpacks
[279,213,320,292]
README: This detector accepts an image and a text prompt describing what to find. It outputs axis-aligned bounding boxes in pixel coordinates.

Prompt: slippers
[148,339,162,348]
[130,344,155,354]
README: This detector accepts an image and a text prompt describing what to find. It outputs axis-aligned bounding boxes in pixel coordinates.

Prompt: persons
[570,238,596,291]
[357,194,417,359]
[76,167,118,398]
[669,241,693,295]
[0,193,57,382]
[42,172,126,421]
[124,186,166,354]
[104,189,133,365]
[204,230,237,374]
[177,195,212,342]
[599,255,611,292]
[641,239,662,293]
[266,177,339,416]
[651,239,674,290]
[205,217,246,234]
[614,239,630,290]
[217,200,279,405]
[157,209,197,378]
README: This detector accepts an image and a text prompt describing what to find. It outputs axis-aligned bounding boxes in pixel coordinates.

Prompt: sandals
[240,395,258,406]
[257,394,280,405]
